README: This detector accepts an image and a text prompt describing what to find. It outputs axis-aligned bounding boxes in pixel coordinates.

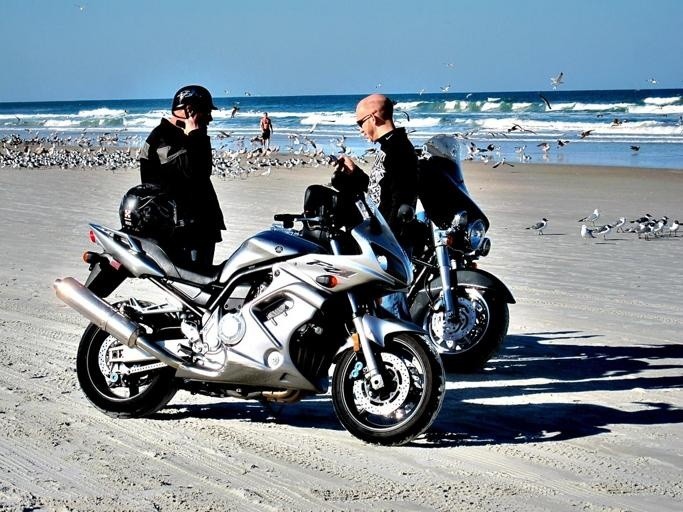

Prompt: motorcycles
[52,156,445,447]
[270,134,516,377]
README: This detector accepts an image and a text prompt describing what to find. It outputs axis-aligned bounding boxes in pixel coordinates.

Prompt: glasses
[357,110,377,128]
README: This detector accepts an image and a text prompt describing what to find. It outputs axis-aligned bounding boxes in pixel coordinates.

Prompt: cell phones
[326,154,338,163]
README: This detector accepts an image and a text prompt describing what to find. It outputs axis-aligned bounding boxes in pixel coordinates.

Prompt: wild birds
[578,207,683,245]
[526,217,548,237]
[644,77,656,87]
[0,70,648,182]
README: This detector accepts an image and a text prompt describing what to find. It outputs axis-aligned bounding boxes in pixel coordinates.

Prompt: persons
[137,89,227,275]
[257,112,273,150]
[332,91,419,228]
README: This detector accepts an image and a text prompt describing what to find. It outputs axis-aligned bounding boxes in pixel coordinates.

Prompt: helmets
[119,183,177,231]
[173,86,218,111]
